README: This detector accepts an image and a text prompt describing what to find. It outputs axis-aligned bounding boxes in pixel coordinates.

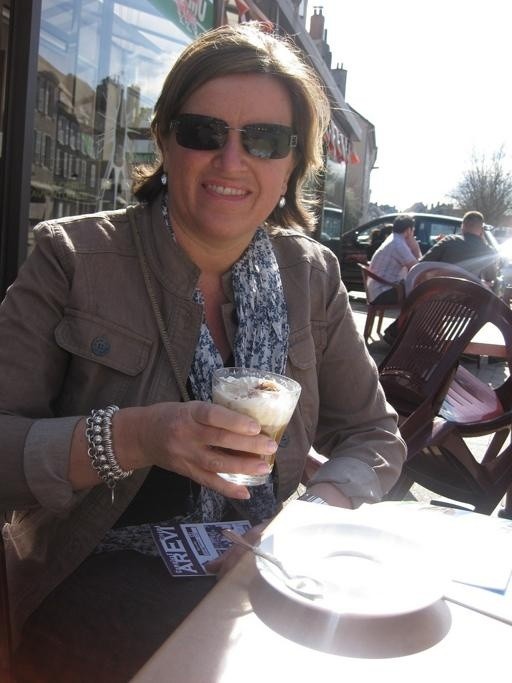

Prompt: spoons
[220,528,326,598]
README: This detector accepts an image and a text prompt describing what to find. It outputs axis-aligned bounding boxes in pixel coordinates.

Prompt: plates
[253,521,450,620]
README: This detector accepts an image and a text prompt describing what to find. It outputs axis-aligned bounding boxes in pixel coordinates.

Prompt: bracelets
[297,491,331,508]
[84,403,134,488]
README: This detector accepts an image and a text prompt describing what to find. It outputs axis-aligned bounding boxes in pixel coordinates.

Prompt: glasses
[161,112,299,161]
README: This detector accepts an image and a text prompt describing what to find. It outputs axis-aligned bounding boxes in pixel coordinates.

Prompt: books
[149,517,253,578]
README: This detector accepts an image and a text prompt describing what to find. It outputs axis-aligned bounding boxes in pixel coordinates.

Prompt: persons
[417,208,501,286]
[364,211,425,308]
[0,21,410,681]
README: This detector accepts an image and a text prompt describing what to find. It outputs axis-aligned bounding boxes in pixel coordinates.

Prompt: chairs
[358,264,406,342]
[378,277,511,516]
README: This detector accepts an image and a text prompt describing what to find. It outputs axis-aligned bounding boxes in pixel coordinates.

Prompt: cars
[321,213,501,291]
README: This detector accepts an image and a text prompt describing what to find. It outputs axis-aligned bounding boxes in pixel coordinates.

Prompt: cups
[209,366,304,488]
